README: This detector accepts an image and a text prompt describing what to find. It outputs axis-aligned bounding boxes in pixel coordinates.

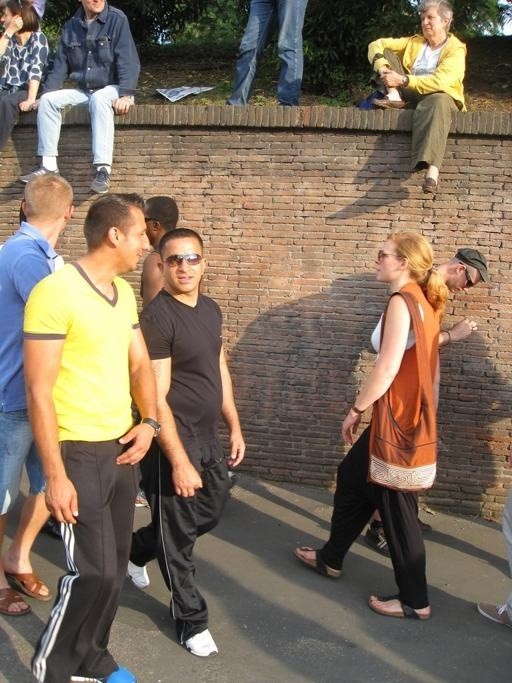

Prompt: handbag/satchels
[366,407,437,491]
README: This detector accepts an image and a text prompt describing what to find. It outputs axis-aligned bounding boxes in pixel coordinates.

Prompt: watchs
[140,418,161,439]
[3,30,11,40]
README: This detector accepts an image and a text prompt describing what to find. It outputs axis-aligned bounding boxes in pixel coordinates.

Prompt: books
[154,82,217,102]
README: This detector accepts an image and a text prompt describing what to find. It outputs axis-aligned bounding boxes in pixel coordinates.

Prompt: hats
[456,248,487,283]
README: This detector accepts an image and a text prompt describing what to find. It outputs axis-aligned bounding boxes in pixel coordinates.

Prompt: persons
[363,246,490,566]
[125,227,247,657]
[367,0,469,194]
[134,196,180,507]
[474,481,511,637]
[1,175,75,615]
[18,0,142,196]
[19,0,46,31]
[24,193,159,683]
[226,0,310,108]
[293,228,450,621]
[1,0,50,153]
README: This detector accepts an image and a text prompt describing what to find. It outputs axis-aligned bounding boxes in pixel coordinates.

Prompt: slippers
[367,595,431,620]
[293,545,341,578]
[0,587,32,616]
[5,568,52,601]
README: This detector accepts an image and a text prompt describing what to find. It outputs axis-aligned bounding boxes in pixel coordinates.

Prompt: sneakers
[185,629,218,656]
[478,602,512,627]
[91,167,110,194]
[70,663,136,683]
[19,167,61,181]
[366,524,391,557]
[128,559,149,588]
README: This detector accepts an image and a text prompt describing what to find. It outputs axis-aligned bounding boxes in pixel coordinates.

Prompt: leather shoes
[422,176,439,192]
[371,97,405,109]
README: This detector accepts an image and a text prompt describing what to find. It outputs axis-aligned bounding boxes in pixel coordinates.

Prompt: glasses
[459,261,473,287]
[378,250,400,261]
[167,255,204,268]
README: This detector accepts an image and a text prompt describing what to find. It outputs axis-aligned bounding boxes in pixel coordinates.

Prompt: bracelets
[351,403,365,415]
[403,75,408,85]
[441,329,451,346]
